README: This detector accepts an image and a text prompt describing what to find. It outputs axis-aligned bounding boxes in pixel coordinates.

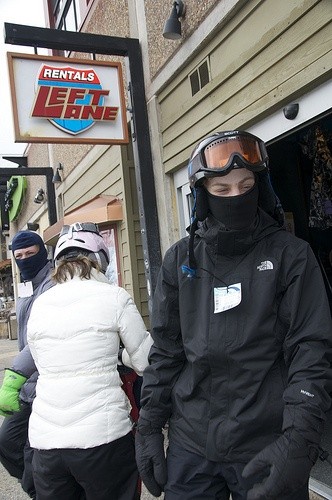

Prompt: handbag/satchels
[116,364,142,426]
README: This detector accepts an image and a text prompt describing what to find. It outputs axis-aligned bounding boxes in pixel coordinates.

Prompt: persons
[0,231,58,500]
[135,129,332,500]
[28,222,154,500]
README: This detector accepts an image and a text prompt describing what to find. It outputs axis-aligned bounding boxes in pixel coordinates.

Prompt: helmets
[187,130,267,187]
[53,222,109,264]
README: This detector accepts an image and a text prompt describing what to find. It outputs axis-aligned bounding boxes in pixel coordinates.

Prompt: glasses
[199,131,267,173]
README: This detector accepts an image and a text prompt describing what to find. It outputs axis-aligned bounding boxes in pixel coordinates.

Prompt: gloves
[242,433,316,500]
[0,368,27,417]
[135,428,167,497]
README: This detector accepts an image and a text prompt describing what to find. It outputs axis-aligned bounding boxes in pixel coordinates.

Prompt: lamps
[8,241,12,250]
[52,163,63,183]
[34,188,45,204]
[162,0,186,40]
[27,223,39,231]
